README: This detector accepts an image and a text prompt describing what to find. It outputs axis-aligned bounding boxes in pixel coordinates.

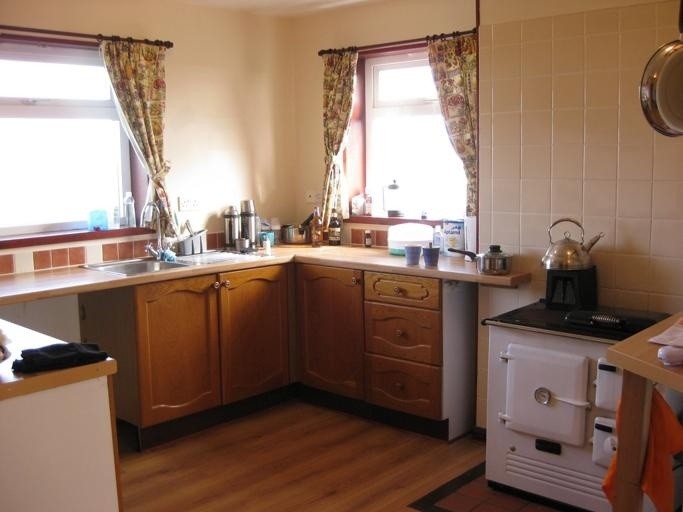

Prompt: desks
[603,308,682,512]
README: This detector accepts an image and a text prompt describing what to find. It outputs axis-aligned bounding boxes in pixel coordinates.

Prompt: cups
[403,244,422,266]
[423,247,441,267]
[234,238,250,251]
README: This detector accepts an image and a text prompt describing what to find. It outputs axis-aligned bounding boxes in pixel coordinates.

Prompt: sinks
[80,256,197,277]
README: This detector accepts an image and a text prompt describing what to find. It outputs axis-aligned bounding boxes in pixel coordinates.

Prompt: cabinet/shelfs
[293,260,364,421]
[364,264,476,442]
[75,261,298,453]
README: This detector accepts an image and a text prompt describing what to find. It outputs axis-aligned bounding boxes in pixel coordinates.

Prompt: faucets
[138,203,163,260]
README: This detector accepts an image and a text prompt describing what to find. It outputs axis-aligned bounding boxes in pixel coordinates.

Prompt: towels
[600,381,683,511]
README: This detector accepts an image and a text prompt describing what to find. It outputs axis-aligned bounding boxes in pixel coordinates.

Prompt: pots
[446,244,513,275]
[637,1,682,138]
[281,212,315,244]
[541,218,605,271]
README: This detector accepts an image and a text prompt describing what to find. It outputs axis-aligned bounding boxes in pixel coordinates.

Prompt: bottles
[240,198,257,249]
[122,191,136,227]
[364,230,372,248]
[327,208,341,246]
[223,205,239,247]
[311,206,323,246]
[387,179,405,217]
